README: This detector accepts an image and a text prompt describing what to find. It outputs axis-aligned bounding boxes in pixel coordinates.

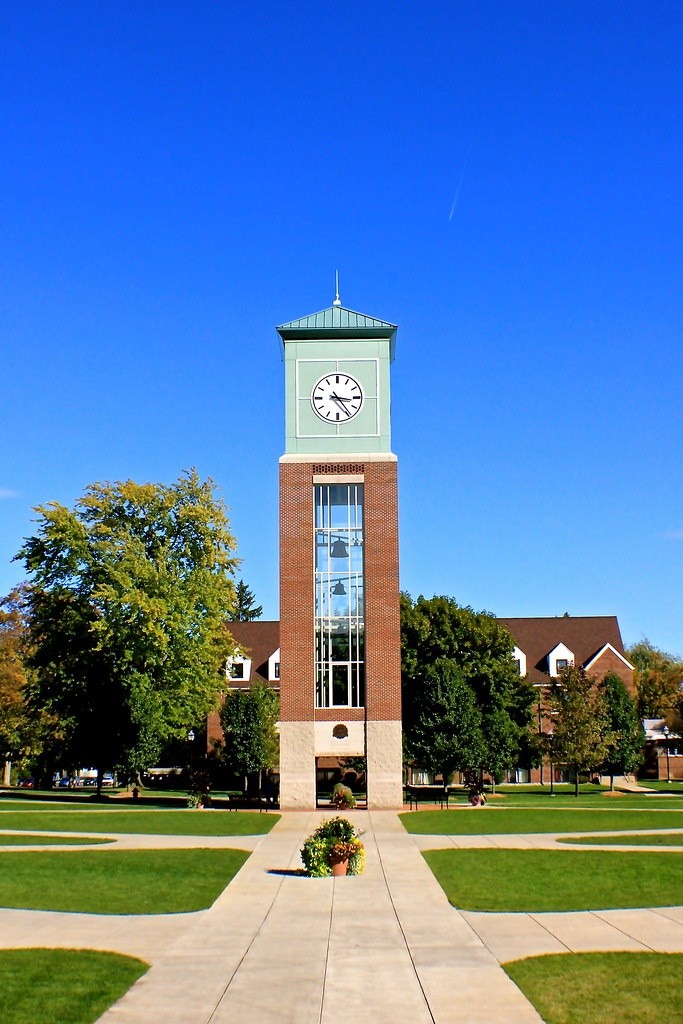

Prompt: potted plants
[329,782,357,810]
[299,815,365,877]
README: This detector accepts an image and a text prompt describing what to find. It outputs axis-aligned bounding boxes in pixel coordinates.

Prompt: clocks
[310,370,365,425]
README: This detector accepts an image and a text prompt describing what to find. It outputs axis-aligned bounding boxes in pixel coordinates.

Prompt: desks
[229,794,268,813]
[409,789,449,810]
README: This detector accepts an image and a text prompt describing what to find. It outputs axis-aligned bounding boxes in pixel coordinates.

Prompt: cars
[102,777,113,786]
[85,778,97,786]
[59,778,70,787]
[16,779,34,787]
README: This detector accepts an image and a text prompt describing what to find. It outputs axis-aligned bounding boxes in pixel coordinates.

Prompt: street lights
[548,733,555,796]
[188,730,195,795]
[663,726,671,783]
